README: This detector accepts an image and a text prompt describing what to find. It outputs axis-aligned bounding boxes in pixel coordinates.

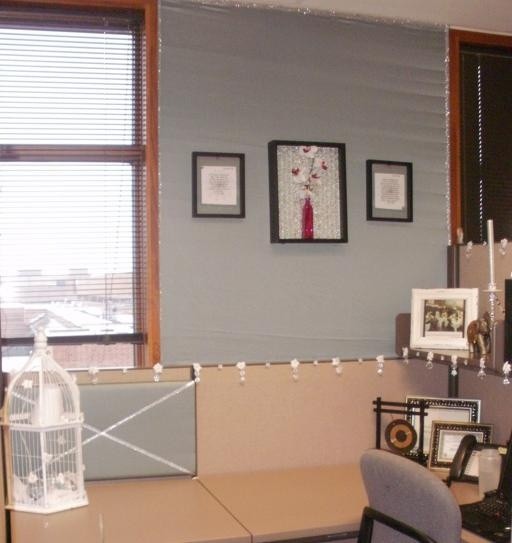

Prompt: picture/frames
[366,158,413,225]
[405,395,483,456]
[267,139,349,245]
[192,152,246,220]
[408,288,479,350]
[431,421,494,469]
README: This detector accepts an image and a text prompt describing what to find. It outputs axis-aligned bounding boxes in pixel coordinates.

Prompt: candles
[487,219,495,286]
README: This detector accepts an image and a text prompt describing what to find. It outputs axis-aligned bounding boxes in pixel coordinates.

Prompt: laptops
[459,432,512,541]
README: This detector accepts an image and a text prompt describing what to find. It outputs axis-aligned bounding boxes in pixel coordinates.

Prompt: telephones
[441,435,507,488]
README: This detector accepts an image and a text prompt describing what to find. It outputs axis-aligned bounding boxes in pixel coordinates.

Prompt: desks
[6,355,512,543]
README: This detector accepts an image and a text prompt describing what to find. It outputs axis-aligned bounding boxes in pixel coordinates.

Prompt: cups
[476,446,502,500]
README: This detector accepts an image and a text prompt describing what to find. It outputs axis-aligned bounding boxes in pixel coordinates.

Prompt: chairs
[359,448,466,543]
